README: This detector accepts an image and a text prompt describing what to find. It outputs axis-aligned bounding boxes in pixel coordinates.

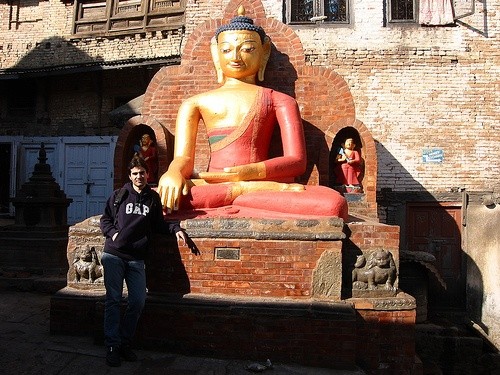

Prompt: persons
[145,1,349,221]
[130,131,158,185]
[336,136,361,193]
[100,156,163,367]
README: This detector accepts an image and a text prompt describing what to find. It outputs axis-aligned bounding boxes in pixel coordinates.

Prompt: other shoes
[106,344,121,367]
[118,341,137,361]
